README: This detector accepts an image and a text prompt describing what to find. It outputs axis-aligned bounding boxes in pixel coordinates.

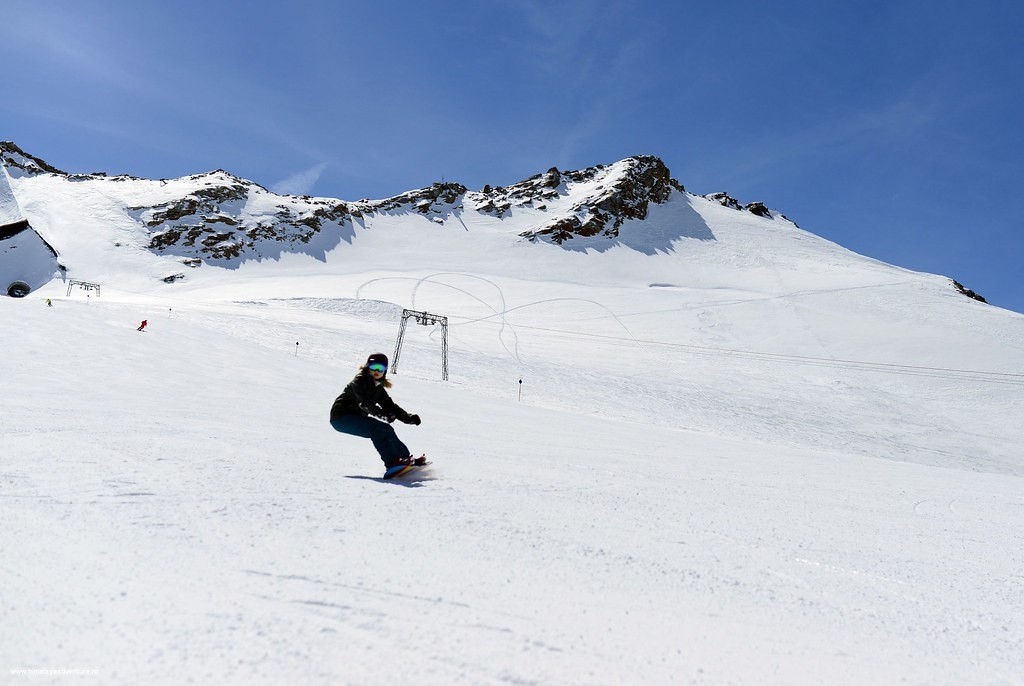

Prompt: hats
[368,354,388,367]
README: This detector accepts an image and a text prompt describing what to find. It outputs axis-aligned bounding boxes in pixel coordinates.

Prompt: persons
[137,319,147,331]
[329,352,426,472]
[45,299,51,306]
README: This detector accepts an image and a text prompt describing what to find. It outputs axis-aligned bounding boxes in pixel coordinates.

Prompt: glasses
[369,364,386,372]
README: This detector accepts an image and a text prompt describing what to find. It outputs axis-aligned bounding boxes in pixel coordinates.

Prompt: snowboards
[383,461,435,480]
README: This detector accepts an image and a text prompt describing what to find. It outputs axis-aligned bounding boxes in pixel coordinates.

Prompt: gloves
[378,409,396,423]
[406,414,420,426]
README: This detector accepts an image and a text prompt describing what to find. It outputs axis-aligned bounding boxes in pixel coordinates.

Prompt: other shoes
[387,455,426,467]
